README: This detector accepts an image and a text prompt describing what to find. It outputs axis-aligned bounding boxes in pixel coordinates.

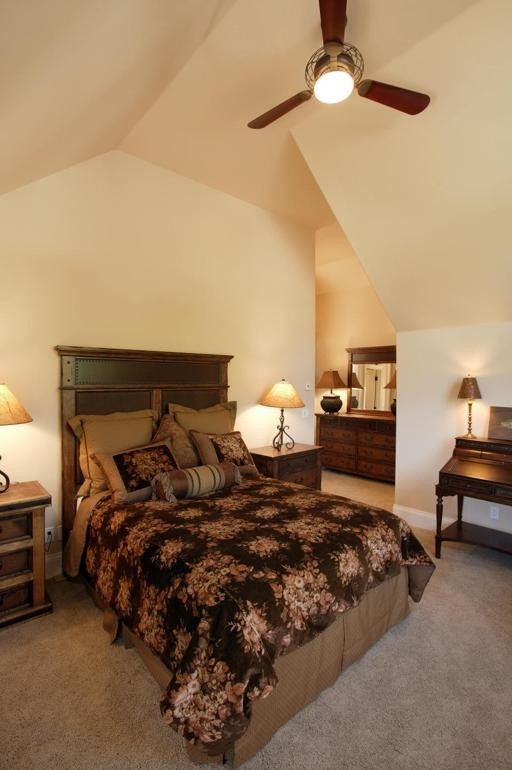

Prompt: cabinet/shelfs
[314,413,395,483]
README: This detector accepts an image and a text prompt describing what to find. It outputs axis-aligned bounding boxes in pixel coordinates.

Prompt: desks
[433,435,512,559]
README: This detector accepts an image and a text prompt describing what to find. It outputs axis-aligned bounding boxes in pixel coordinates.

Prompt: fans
[249,1,430,129]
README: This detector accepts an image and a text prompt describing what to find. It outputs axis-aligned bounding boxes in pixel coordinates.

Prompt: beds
[54,346,408,770]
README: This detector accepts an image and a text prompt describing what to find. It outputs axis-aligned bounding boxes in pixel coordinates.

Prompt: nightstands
[0,481,52,628]
[248,442,325,492]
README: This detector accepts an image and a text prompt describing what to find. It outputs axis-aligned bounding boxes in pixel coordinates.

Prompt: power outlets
[45,527,55,544]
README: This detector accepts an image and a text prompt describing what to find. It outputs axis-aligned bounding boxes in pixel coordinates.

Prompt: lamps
[260,378,305,451]
[351,373,364,408]
[0,383,34,492]
[457,374,482,439]
[316,369,348,413]
[314,71,354,104]
[383,371,396,416]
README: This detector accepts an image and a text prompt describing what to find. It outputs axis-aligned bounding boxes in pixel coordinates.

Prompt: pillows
[66,400,259,503]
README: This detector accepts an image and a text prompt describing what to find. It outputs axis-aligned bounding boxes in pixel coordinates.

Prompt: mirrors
[344,345,397,417]
